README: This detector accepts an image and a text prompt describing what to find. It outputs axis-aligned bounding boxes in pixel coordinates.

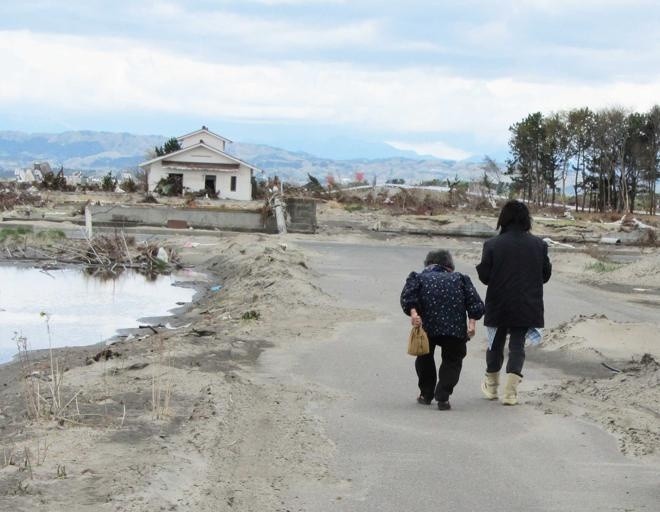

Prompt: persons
[398,247,485,410]
[476,200,554,404]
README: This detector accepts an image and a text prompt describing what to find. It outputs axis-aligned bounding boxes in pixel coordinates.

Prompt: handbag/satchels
[407,326,430,355]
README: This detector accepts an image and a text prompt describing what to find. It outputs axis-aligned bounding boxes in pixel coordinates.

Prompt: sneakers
[417,395,450,409]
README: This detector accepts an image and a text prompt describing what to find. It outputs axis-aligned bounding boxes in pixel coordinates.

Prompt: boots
[501,373,525,405]
[480,369,501,400]
